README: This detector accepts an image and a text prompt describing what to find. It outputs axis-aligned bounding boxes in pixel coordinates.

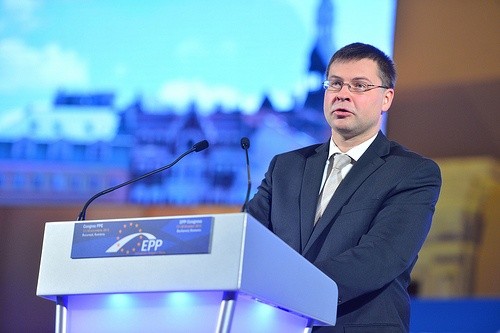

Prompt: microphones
[241,137,251,212]
[76,141,209,220]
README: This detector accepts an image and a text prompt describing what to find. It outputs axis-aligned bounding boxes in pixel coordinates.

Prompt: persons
[245,42,443,333]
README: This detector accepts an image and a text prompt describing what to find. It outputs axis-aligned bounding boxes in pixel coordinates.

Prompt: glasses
[322,79,390,93]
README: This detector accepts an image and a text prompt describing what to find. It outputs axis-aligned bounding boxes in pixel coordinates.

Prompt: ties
[314,154,351,222]
